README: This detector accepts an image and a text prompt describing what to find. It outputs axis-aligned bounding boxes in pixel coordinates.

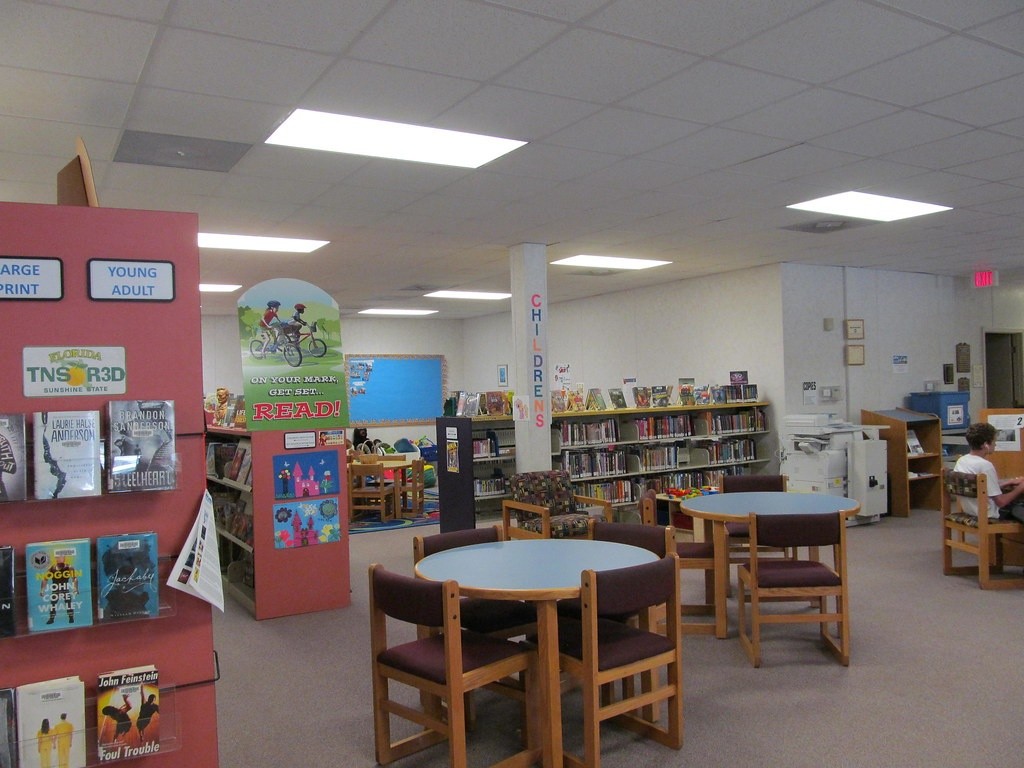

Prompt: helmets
[267,300,281,307]
[295,304,307,310]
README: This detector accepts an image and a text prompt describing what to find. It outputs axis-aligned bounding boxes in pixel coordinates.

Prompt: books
[636,466,745,494]
[206,437,252,485]
[0,662,160,768]
[712,411,766,435]
[33,410,102,500]
[474,478,506,497]
[907,429,923,453]
[212,489,253,547]
[105,400,176,493]
[572,477,635,508]
[473,437,495,460]
[486,431,499,456]
[450,390,514,416]
[226,395,246,429]
[552,417,619,446]
[0,413,27,502]
[0,545,17,638]
[561,446,676,479]
[26,532,160,634]
[626,414,696,441]
[550,382,759,412]
[696,438,757,465]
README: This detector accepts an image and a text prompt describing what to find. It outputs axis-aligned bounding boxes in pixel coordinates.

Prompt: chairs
[346,454,425,522]
[528,552,682,768]
[736,510,852,670]
[941,466,1024,590]
[411,468,790,642]
[368,563,543,767]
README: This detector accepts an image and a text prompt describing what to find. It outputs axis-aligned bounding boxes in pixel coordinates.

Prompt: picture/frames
[497,364,508,387]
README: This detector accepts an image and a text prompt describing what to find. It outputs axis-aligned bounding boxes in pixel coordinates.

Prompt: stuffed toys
[354,427,373,452]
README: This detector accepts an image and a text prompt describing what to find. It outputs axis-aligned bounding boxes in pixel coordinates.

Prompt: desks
[346,460,414,519]
[413,538,663,768]
[678,492,861,641]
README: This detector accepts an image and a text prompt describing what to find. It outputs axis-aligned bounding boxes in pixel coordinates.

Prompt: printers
[779,412,891,527]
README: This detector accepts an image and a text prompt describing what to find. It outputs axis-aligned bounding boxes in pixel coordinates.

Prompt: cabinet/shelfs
[550,402,770,517]
[435,415,516,533]
[861,406,943,519]
[206,424,350,621]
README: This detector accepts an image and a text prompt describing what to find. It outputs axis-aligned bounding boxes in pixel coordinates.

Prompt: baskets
[419,437,438,460]
[347,455,353,463]
[359,440,378,464]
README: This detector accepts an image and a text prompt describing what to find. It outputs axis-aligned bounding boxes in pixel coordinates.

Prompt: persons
[257,300,280,359]
[952,423,1024,521]
[216,387,229,422]
[288,304,307,350]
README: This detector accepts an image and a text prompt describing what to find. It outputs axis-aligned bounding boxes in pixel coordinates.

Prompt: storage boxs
[910,391,970,429]
